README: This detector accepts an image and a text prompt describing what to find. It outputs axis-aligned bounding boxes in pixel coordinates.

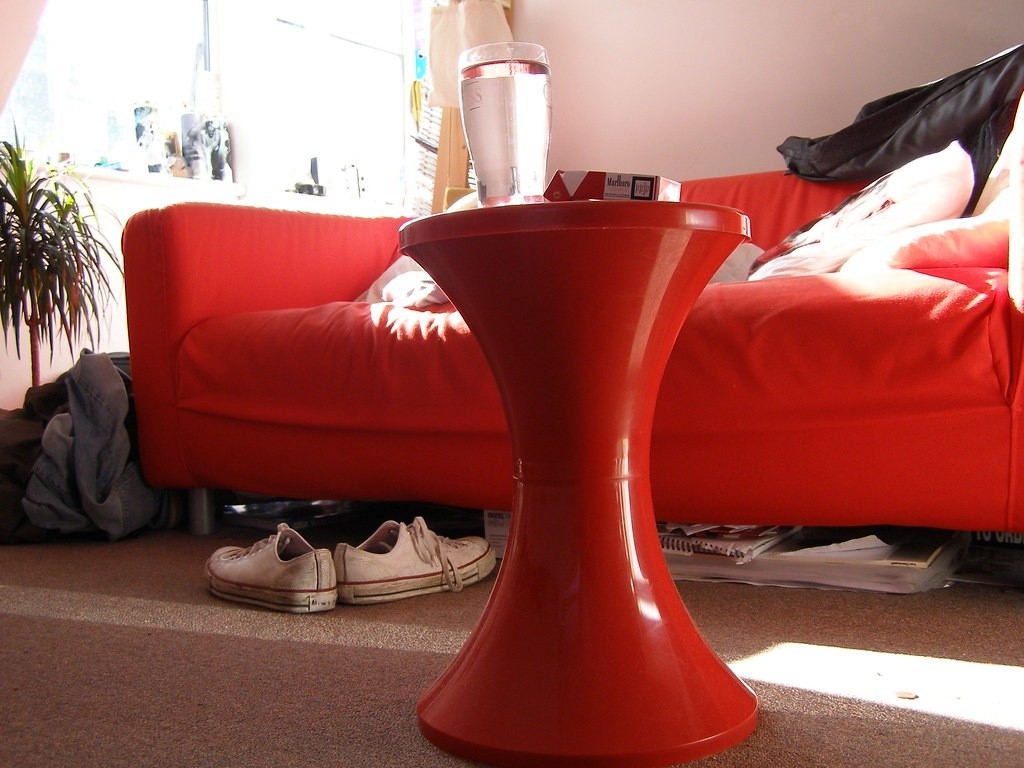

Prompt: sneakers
[333,517,496,604]
[203,523,338,613]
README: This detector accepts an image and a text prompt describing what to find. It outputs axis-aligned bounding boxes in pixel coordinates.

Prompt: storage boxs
[482,510,511,560]
[542,168,681,202]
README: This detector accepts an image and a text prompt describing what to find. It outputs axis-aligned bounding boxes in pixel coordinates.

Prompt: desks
[394,199,761,768]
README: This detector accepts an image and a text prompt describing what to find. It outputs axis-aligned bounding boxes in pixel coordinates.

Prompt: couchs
[122,168,1024,536]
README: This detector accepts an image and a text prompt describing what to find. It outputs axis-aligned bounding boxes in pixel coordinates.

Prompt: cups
[459,41,552,208]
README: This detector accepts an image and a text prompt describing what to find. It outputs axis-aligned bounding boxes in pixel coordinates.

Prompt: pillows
[752,138,975,277]
[839,131,1009,269]
[354,191,478,308]
